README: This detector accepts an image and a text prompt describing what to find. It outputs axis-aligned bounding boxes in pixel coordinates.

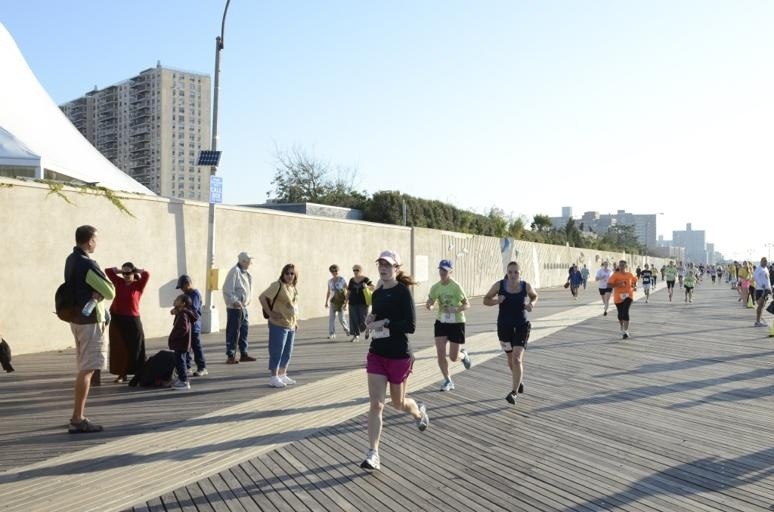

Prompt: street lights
[764,242,774,263]
[644,212,665,267]
[746,248,755,257]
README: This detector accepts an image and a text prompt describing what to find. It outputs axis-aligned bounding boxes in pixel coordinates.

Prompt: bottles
[81,298,98,317]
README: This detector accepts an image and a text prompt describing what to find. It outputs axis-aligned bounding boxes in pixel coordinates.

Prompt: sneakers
[226,357,239,364]
[193,367,208,376]
[518,383,524,393]
[187,368,193,373]
[753,321,768,328]
[171,379,191,390]
[68,418,103,434]
[239,355,256,361]
[359,448,382,473]
[415,400,430,433]
[505,390,519,405]
[441,381,455,392]
[621,330,630,340]
[460,348,471,370]
[270,376,287,387]
[279,375,296,385]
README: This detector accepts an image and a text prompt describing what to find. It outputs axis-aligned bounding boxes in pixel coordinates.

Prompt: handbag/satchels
[363,287,373,305]
[334,293,346,306]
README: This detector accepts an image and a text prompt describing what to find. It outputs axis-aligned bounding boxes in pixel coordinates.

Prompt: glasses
[286,271,294,276]
[122,271,133,276]
[353,269,360,272]
[331,270,338,273]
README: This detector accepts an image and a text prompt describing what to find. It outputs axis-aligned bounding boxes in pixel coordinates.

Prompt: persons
[359,250,430,472]
[171,274,208,376]
[63,224,116,434]
[345,264,375,343]
[168,294,193,390]
[425,259,471,391]
[222,252,257,364]
[483,261,538,405]
[104,261,149,384]
[567,257,774,339]
[258,263,299,387]
[325,264,351,340]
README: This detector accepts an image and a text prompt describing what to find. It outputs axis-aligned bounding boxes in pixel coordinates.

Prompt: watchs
[383,318,388,327]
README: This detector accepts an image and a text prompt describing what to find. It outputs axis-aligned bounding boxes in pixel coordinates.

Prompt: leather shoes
[112,374,129,383]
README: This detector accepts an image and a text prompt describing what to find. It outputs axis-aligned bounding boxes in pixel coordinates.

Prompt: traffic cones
[746,293,754,308]
[768,323,774,338]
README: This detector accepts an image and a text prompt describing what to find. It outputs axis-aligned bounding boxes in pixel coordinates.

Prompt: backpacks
[54,280,86,322]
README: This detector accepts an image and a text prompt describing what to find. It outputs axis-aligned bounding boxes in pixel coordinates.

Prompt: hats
[376,249,403,267]
[175,274,193,289]
[437,259,455,272]
[238,252,255,265]
